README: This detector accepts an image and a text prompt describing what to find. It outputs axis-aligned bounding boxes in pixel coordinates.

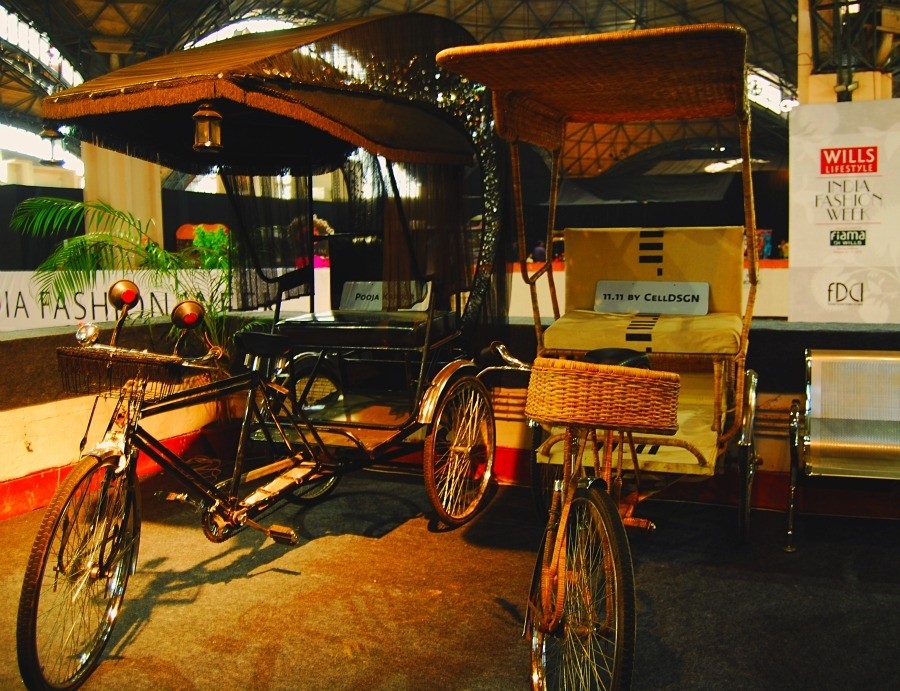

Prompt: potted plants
[9,197,266,459]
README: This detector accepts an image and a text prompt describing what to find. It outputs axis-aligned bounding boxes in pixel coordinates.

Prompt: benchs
[541,226,747,430]
[784,348,900,551]
[273,222,473,427]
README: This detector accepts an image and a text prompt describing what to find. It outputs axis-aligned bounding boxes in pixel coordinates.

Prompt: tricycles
[431,23,769,691]
[11,14,518,691]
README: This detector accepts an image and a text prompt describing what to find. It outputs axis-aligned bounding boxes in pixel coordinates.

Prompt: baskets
[524,357,680,436]
[56,345,182,403]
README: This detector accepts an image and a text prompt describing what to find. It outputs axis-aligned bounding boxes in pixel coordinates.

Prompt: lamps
[192,100,224,154]
